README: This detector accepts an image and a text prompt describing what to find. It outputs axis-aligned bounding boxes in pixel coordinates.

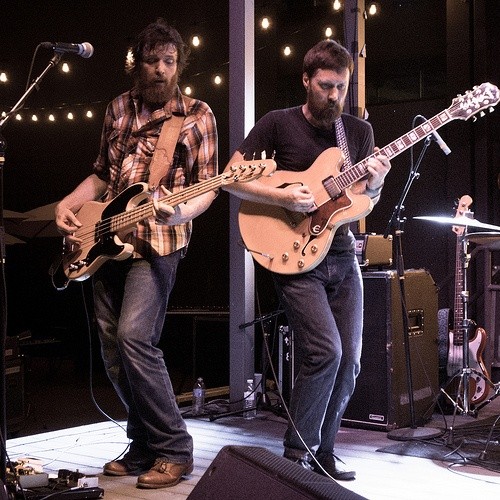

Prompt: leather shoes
[103,450,156,476]
[136,459,194,488]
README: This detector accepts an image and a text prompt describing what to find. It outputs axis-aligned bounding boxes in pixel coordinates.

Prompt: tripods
[209,308,290,425]
[422,226,500,445]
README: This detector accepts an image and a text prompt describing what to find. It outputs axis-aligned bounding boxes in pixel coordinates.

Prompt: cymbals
[413,212,500,249]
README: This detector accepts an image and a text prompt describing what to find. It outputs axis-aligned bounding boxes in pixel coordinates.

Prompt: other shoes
[289,457,312,471]
[312,466,357,480]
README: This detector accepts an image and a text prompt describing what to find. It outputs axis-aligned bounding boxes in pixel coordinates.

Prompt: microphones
[41,41,94,58]
[421,116,451,155]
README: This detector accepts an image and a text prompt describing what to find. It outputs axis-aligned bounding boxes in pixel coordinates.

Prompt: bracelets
[365,181,385,195]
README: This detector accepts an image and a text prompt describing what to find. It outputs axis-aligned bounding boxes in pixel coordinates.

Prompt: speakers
[186,445,367,500]
[289,268,439,432]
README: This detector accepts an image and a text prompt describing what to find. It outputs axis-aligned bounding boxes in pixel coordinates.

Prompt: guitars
[237,81,500,274]
[442,192,492,407]
[36,147,276,289]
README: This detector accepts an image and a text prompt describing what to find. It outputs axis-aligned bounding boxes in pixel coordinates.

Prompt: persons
[220,39,393,481]
[53,23,220,488]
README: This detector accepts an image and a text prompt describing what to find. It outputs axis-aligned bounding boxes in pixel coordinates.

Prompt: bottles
[192,377,205,416]
[243,379,257,420]
